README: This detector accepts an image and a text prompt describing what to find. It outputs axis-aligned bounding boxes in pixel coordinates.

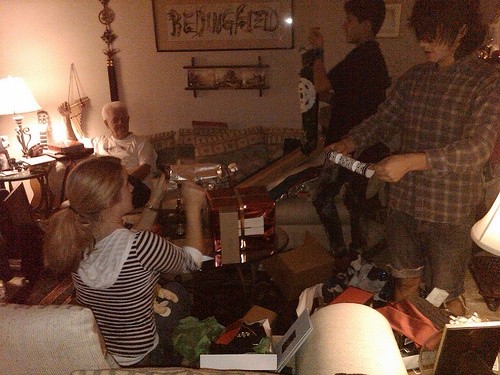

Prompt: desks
[0,167,52,210]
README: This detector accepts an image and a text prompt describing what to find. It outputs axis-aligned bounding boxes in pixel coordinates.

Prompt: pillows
[0,302,123,374]
[193,124,268,165]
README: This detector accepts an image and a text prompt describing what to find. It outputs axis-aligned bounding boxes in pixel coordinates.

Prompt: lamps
[0,75,42,157]
[470,190,500,256]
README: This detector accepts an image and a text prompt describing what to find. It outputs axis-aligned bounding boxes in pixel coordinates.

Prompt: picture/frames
[376,4,402,38]
[151,0,294,52]
[0,150,13,170]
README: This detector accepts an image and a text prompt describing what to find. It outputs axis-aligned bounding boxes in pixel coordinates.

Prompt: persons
[44,156,205,368]
[324,0,500,316]
[309,0,390,299]
[59,100,158,210]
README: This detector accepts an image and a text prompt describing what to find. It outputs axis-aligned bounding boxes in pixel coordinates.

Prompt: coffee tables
[128,209,289,304]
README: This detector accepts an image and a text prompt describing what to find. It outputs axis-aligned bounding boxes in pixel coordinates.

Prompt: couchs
[72,303,412,375]
[32,128,388,252]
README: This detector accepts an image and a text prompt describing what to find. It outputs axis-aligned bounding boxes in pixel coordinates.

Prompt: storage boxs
[274,229,335,301]
[206,188,277,263]
[199,305,277,371]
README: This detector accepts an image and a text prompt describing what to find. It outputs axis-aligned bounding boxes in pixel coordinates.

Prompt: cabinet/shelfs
[183,56,270,98]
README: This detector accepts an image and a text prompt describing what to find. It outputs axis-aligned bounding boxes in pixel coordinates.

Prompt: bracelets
[144,204,158,212]
